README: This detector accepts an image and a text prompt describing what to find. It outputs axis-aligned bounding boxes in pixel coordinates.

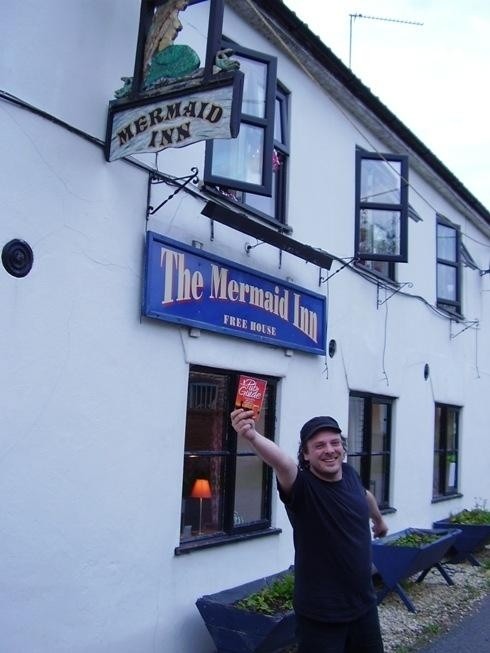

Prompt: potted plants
[370,494,489,615]
[194,563,299,653]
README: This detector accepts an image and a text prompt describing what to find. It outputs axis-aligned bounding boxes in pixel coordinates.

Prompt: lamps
[190,477,213,536]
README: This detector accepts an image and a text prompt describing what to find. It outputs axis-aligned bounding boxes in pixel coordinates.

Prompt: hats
[300,415,341,442]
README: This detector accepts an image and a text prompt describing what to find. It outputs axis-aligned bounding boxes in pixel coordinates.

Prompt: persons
[230,409,388,653]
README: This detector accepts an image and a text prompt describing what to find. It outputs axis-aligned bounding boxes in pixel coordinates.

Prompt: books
[234,375,267,422]
